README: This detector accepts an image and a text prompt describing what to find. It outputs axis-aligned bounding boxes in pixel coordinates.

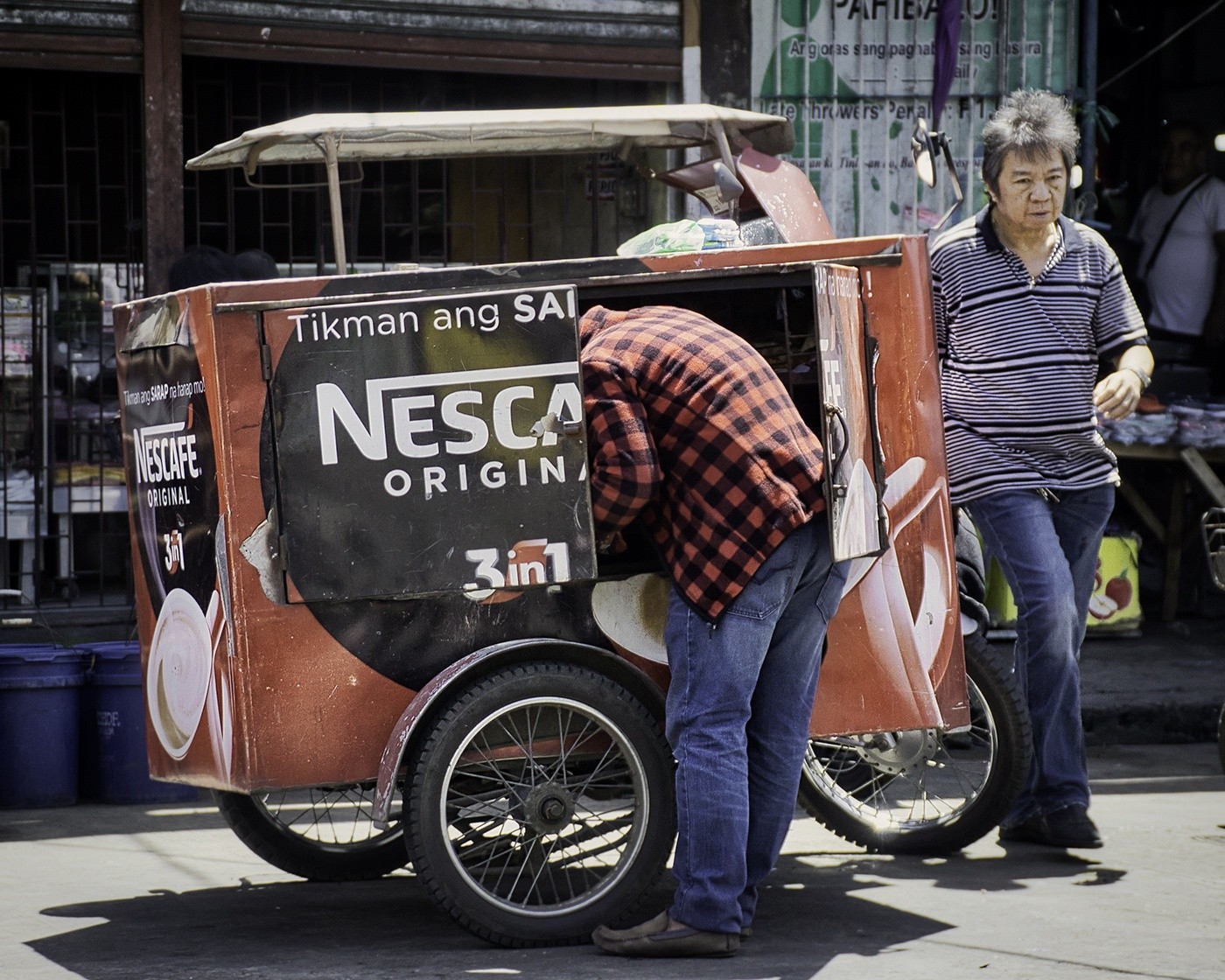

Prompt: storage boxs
[982,536,1142,630]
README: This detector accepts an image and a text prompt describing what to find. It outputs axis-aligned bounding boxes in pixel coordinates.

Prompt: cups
[145,587,212,759]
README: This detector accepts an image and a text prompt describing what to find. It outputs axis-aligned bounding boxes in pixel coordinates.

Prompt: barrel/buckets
[69,641,198,804]
[0,618,95,811]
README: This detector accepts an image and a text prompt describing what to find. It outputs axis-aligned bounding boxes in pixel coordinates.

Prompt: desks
[1096,434,1225,630]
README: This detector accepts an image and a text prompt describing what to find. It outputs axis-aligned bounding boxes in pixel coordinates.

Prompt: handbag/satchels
[1130,279,1152,324]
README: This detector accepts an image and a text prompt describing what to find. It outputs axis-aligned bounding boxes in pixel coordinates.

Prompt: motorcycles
[109,102,1037,952]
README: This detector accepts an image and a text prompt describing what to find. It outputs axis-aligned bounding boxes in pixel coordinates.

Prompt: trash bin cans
[0,643,84,807]
[75,640,198,804]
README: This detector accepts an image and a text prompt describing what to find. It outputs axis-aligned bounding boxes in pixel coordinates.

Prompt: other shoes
[998,816,1049,846]
[1048,805,1102,848]
[591,908,743,958]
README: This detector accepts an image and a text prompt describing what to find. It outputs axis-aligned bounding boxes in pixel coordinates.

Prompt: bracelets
[1123,365,1151,395]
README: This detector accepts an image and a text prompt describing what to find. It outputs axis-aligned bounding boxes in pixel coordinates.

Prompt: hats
[593,572,674,666]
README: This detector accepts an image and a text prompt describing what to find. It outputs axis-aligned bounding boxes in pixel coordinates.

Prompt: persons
[577,305,852,957]
[169,249,280,290]
[930,90,1153,853]
[1126,127,1225,356]
